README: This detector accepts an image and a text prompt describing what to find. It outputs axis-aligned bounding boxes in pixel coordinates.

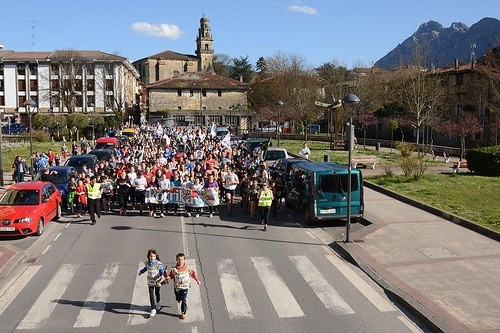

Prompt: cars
[2,123,25,135]
[256,125,282,133]
[0,181,62,236]
[37,128,139,208]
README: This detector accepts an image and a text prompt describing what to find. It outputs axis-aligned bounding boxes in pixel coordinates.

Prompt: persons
[138,249,165,318]
[299,143,310,160]
[162,253,200,319]
[11,122,279,233]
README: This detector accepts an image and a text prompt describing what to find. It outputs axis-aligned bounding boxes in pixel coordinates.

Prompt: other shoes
[92,221,96,225]
[161,213,164,217]
[182,311,187,319]
[98,214,100,218]
[149,212,156,216]
[149,309,156,317]
[156,300,161,310]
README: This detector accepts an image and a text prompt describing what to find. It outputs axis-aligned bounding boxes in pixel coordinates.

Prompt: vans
[305,124,320,135]
[285,162,364,225]
[217,127,309,199]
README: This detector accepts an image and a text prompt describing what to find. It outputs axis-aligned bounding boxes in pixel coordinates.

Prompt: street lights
[238,102,242,136]
[23,99,36,180]
[342,93,360,243]
[229,106,232,125]
[276,100,284,147]
[232,104,237,126]
[88,102,95,146]
